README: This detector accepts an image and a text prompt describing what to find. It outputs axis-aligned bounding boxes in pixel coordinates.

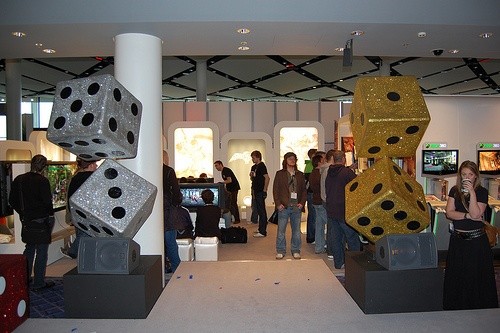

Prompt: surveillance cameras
[433,50,444,57]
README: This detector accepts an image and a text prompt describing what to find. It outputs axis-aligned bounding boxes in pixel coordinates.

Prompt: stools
[195,236,219,261]
[176,238,194,262]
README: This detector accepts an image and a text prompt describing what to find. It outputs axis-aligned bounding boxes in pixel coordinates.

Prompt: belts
[455,229,484,239]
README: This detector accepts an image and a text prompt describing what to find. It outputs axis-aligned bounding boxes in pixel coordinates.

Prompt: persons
[163,150,181,272]
[195,190,222,237]
[9,154,55,277]
[59,153,96,261]
[444,160,499,310]
[273,152,307,259]
[214,160,241,224]
[176,192,194,239]
[247,151,271,237]
[181,173,207,183]
[304,149,360,269]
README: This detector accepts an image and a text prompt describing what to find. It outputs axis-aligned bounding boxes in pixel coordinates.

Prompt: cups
[462,179,471,192]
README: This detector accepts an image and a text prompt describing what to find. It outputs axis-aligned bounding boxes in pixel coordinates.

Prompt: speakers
[375,231,437,272]
[75,238,139,275]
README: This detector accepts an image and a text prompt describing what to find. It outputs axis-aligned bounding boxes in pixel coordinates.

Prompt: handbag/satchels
[21,224,30,243]
[220,226,247,244]
[168,203,192,230]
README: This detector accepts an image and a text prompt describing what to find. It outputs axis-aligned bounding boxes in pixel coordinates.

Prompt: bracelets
[464,212,467,219]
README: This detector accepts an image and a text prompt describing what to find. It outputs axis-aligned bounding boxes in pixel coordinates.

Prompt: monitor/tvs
[421,149,459,176]
[477,149,500,175]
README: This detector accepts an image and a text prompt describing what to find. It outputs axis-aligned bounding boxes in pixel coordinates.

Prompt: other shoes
[247,220,255,224]
[254,228,259,232]
[276,254,284,259]
[292,252,301,259]
[254,233,265,237]
[312,242,315,244]
[33,281,55,291]
[328,256,334,260]
[60,247,73,260]
